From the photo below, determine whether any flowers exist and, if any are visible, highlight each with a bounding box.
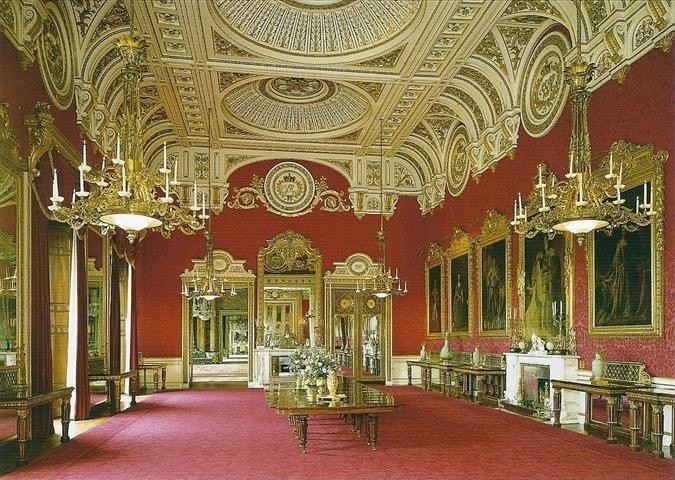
[285,334,344,380]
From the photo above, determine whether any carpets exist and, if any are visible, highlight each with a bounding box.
[1,388,675,480]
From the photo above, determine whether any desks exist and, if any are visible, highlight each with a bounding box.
[139,361,168,393]
[406,359,507,404]
[91,369,139,413]
[550,378,675,460]
[0,384,76,467]
[260,374,407,454]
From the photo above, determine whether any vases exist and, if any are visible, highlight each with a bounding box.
[440,339,455,360]
[591,351,606,380]
[419,345,427,359]
[325,373,339,397]
[472,347,481,367]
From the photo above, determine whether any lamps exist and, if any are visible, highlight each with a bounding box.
[353,116,410,300]
[47,0,209,244]
[178,106,240,302]
[507,0,659,240]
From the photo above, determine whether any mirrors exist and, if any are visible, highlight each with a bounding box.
[178,229,393,381]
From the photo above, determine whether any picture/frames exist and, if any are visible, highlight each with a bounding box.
[585,138,669,339]
[444,226,476,339]
[516,163,577,342]
[475,209,514,341]
[425,240,447,340]
